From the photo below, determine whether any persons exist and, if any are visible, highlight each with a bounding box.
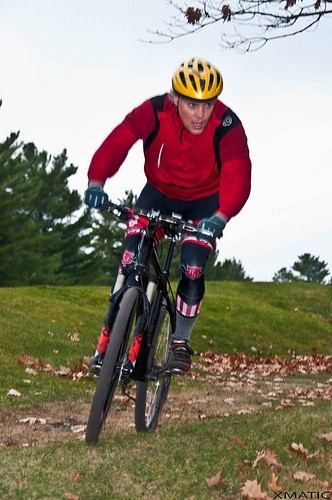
[85,57,251,376]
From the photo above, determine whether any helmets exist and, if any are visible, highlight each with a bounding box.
[172,57,222,103]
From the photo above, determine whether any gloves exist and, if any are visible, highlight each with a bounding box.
[196,215,226,240]
[84,185,108,208]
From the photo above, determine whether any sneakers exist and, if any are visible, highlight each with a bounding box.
[91,351,105,373]
[168,339,193,374]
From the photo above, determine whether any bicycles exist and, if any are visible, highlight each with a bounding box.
[81,197,218,448]
